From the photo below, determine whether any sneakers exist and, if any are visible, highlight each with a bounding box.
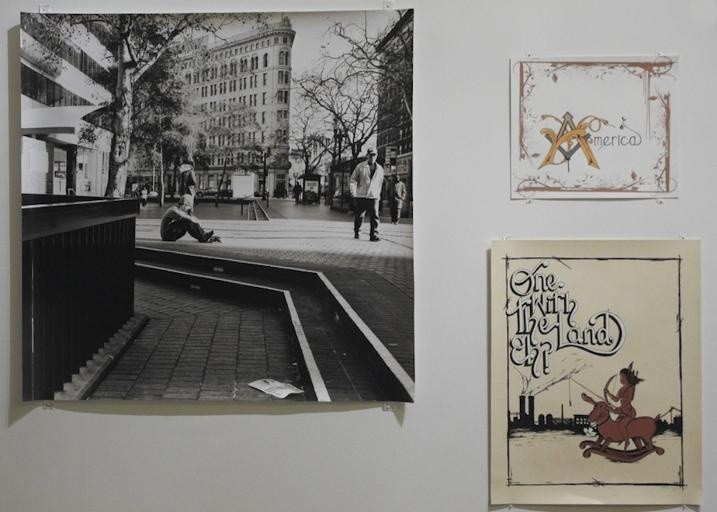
[370,238,380,241]
[354,234,359,238]
[199,230,213,243]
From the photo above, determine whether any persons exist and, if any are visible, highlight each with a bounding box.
[293,182,301,203]
[160,194,222,244]
[605,367,635,450]
[387,175,406,224]
[349,147,383,240]
[175,160,195,214]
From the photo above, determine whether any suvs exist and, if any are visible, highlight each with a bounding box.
[134,181,161,197]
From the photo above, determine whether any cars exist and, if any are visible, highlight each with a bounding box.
[198,186,232,198]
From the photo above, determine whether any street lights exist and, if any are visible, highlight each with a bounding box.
[153,114,167,206]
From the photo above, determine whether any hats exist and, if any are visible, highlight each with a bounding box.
[367,148,377,154]
